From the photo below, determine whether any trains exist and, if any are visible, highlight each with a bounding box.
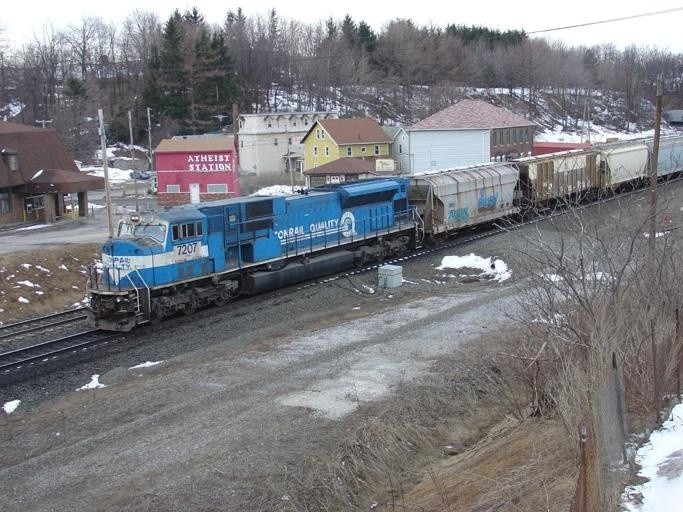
[81,130,683,332]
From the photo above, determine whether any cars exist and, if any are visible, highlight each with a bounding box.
[129,169,150,180]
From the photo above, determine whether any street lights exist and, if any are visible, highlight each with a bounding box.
[35,117,54,128]
